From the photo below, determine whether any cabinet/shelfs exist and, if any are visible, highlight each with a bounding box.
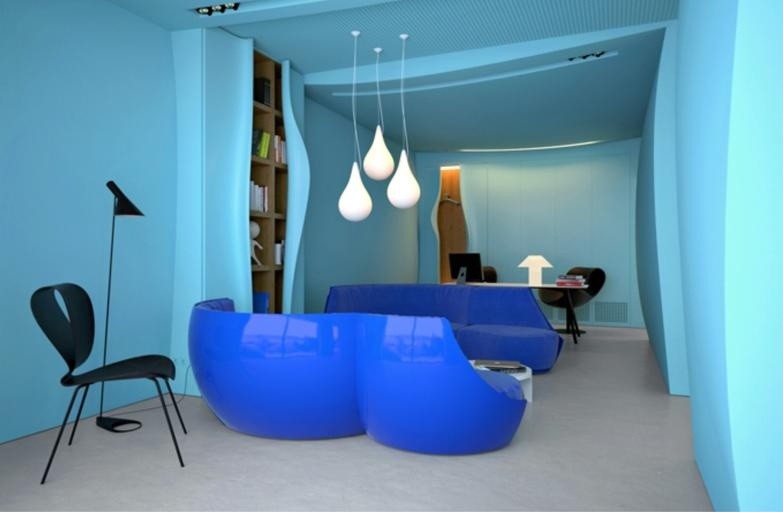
[249,50,288,313]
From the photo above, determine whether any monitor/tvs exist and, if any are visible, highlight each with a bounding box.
[448,252,482,285]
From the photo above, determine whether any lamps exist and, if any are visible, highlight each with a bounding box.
[96,180,145,435]
[336,28,422,225]
[517,254,553,285]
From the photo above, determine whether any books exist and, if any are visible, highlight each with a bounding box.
[249,179,268,213]
[252,128,286,164]
[555,274,587,288]
[471,358,526,375]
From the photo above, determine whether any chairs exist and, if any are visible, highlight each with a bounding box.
[30,283,188,489]
[538,268,606,334]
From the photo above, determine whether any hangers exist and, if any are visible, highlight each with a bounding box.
[435,191,461,207]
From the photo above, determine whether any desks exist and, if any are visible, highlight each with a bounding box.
[443,282,588,346]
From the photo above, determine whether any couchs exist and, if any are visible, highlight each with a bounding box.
[323,284,561,372]
[189,297,363,439]
[354,312,527,456]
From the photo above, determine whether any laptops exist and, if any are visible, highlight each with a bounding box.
[474,359,521,370]
